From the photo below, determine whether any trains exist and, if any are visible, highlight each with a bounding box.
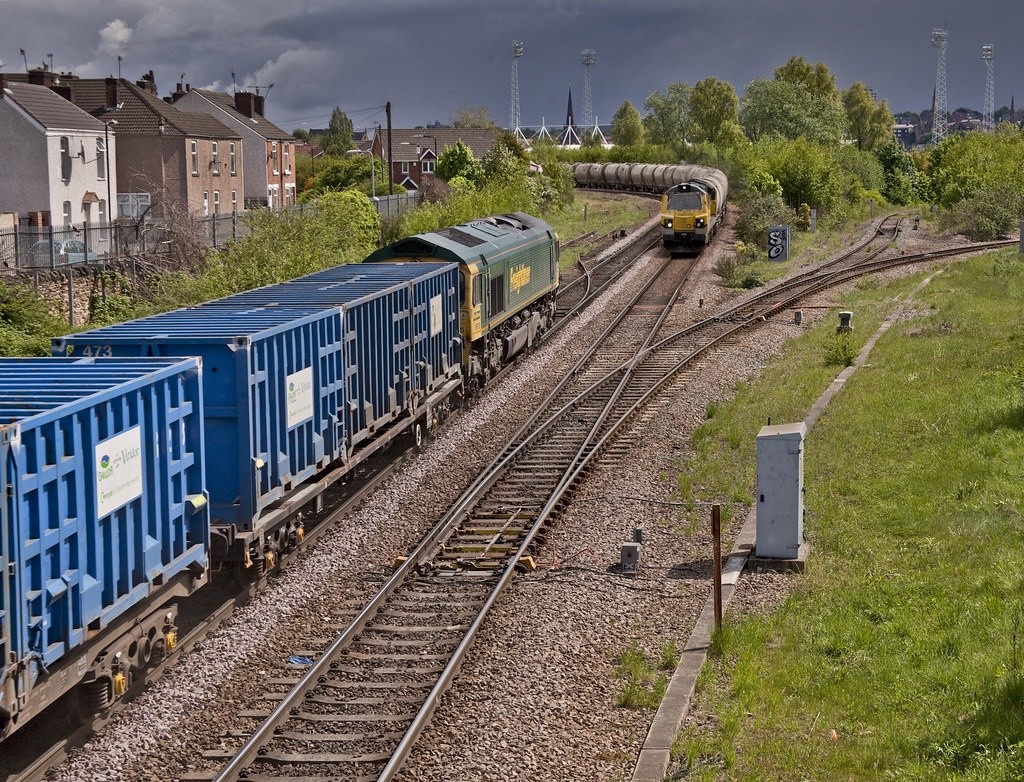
[0,210,562,744]
[569,161,729,256]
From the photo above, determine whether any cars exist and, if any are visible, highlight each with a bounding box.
[25,238,98,279]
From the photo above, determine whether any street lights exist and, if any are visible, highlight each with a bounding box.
[401,142,420,188]
[411,134,438,179]
[304,144,314,190]
[347,150,379,206]
[105,119,118,263]
